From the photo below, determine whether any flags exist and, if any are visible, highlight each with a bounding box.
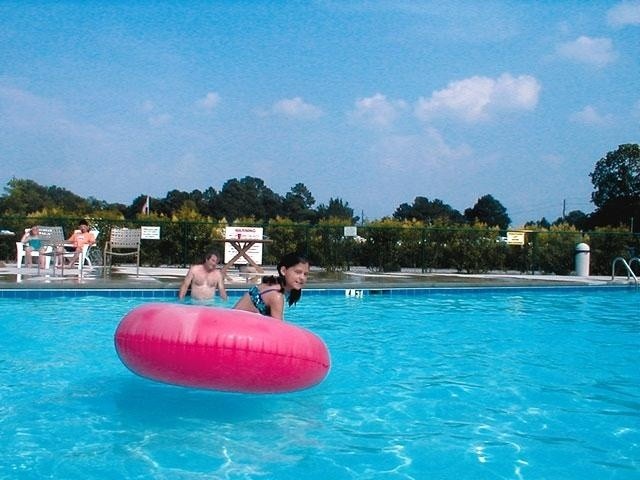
[142,195,149,214]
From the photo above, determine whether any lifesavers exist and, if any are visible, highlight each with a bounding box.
[115,303,330,392]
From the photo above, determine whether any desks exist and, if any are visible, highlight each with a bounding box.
[40,241,73,278]
[212,239,273,273]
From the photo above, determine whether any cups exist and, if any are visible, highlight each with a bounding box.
[237,233,241,239]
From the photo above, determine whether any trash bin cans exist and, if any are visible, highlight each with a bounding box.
[574,243,591,277]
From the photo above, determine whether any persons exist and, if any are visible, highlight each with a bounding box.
[231,251,312,322]
[55,220,96,269]
[20,224,48,269]
[179,251,227,301]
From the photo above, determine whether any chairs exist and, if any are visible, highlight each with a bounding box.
[58,230,100,269]
[15,229,53,269]
[104,227,142,278]
